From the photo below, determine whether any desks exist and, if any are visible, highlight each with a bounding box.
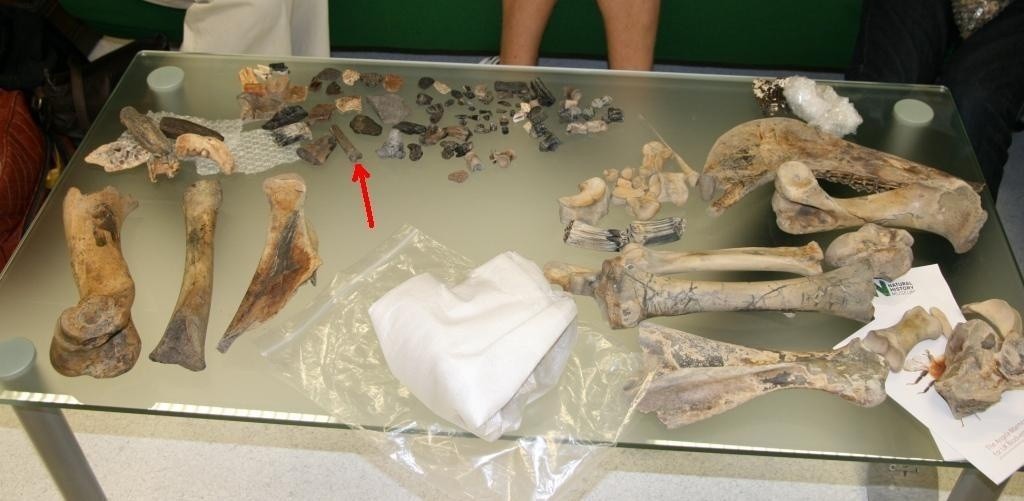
[0,50,1024,501]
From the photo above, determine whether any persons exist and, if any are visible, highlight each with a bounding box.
[142,1,331,57]
[477,0,661,72]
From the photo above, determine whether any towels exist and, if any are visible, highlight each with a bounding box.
[370,254,577,440]
